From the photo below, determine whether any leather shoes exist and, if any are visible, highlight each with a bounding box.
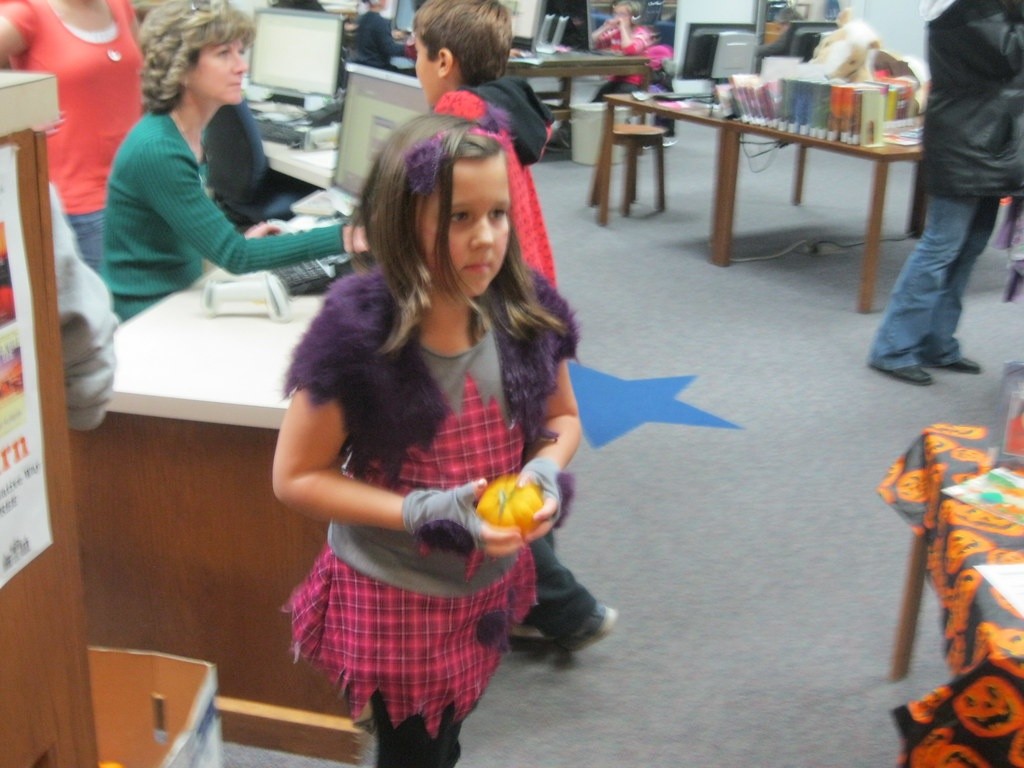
[870,364,933,386]
[938,358,980,374]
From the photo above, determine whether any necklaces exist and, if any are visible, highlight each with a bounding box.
[107,47,121,61]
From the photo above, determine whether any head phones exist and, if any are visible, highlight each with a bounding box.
[631,12,641,24]
[370,0,379,5]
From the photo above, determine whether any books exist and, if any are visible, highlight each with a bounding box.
[714,73,917,148]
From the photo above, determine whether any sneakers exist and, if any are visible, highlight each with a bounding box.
[557,603,619,651]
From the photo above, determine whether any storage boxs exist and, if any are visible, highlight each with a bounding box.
[85,643,219,768]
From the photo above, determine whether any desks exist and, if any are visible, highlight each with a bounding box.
[66,213,375,768]
[598,94,929,314]
[876,421,1024,768]
[339,22,653,156]
[234,74,343,192]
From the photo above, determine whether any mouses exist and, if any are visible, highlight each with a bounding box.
[631,90,650,100]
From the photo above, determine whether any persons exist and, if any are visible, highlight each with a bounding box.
[411,0,619,658]
[356,0,407,72]
[0,0,143,276]
[99,0,367,323]
[759,6,803,57]
[270,112,583,768]
[591,0,657,102]
[864,0,1024,385]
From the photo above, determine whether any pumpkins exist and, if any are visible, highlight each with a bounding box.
[477,472,545,537]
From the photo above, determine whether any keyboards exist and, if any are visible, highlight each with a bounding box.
[252,118,308,149]
[592,50,615,56]
[269,257,335,295]
[654,94,713,101]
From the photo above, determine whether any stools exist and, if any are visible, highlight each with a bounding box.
[588,123,668,219]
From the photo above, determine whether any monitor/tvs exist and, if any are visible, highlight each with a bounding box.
[331,63,430,208]
[537,0,592,54]
[785,20,839,61]
[677,22,756,89]
[249,7,344,108]
[393,0,418,35]
